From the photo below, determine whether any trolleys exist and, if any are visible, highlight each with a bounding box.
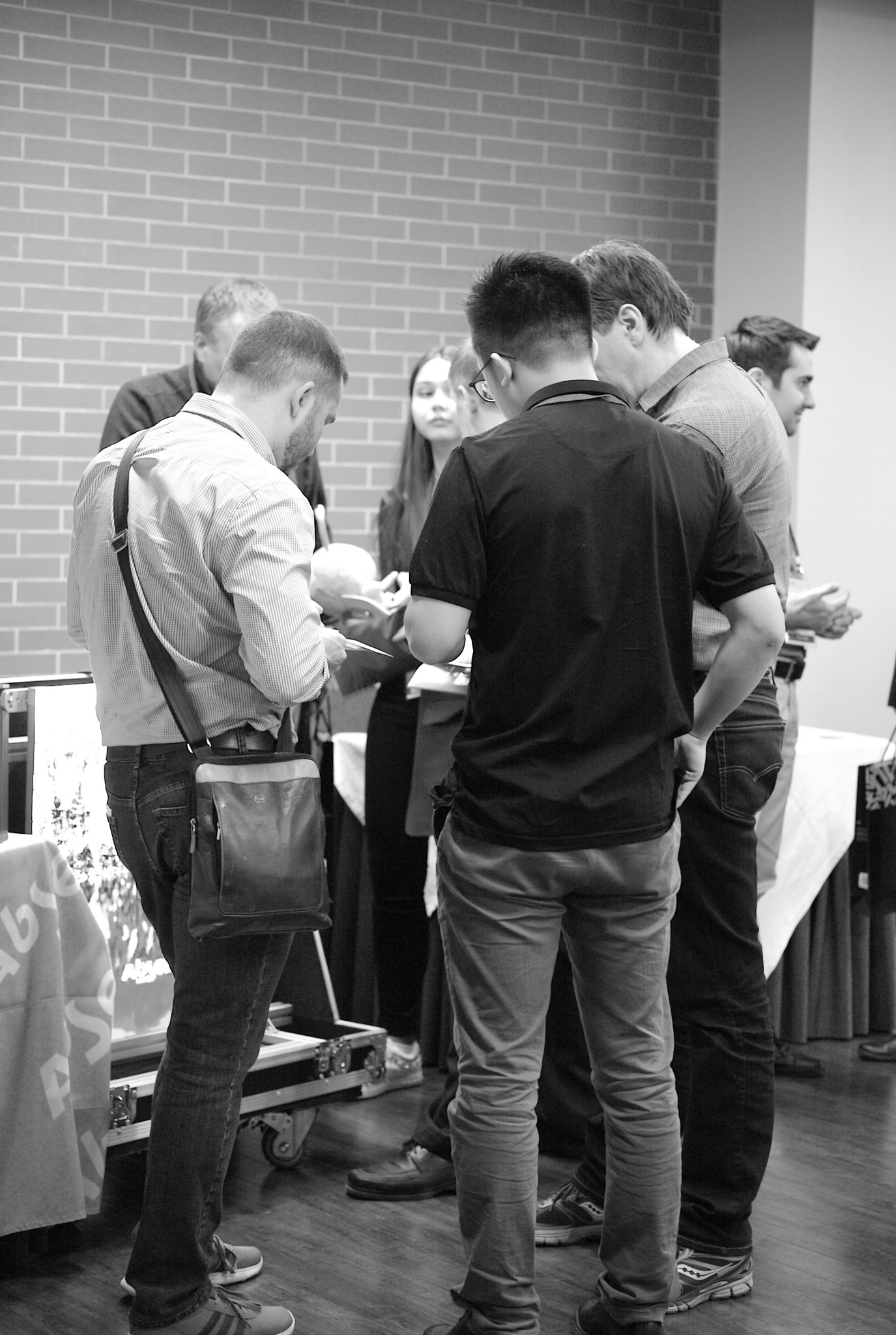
[0,672,387,1252]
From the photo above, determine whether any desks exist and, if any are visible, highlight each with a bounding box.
[329,727,896,1076]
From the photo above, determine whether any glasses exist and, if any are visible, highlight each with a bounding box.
[468,352,517,403]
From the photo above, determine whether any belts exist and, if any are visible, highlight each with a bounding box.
[774,660,805,680]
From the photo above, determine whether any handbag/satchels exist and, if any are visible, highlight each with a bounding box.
[849,729,896,920]
[183,747,332,938]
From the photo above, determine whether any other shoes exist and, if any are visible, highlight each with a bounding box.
[358,1039,423,1101]
[422,1317,470,1335]
[576,1295,667,1335]
[776,1053,825,1077]
[859,1032,896,1061]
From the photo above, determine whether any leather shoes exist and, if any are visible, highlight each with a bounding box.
[347,1140,458,1202]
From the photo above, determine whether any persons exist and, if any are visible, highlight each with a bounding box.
[534,241,790,1315]
[338,344,593,1204]
[711,311,871,1080]
[856,653,896,1061]
[96,267,362,1023]
[68,309,364,1335]
[396,250,790,1335]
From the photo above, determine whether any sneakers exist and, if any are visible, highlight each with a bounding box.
[535,1180,603,1244]
[121,1233,264,1296]
[130,1285,296,1334]
[666,1246,754,1316]
